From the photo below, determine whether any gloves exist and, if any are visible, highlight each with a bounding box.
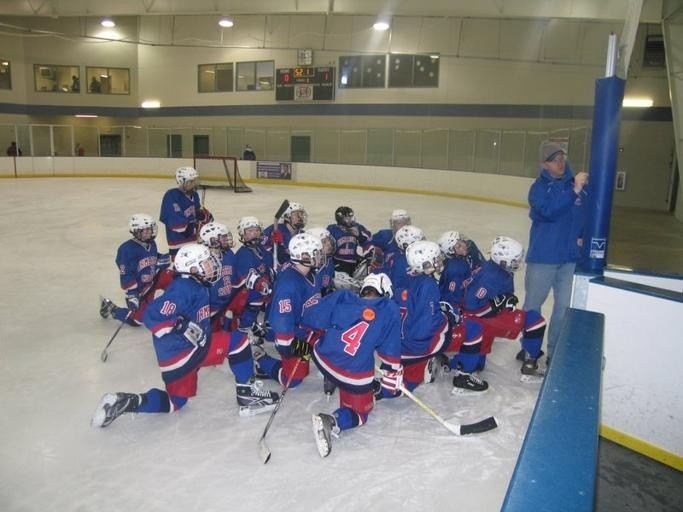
[488,292,520,313]
[186,222,203,234]
[294,338,314,362]
[441,308,465,328]
[377,363,405,394]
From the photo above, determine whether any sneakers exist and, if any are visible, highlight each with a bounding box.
[238,323,272,346]
[423,353,448,384]
[451,371,489,398]
[126,290,142,309]
[97,296,114,318]
[310,411,340,459]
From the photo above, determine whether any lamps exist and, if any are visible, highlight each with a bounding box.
[218,16,233,27]
[373,15,390,30]
[101,16,115,27]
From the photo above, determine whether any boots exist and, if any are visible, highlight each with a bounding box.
[232,380,281,417]
[322,375,337,402]
[89,388,142,429]
[250,344,267,378]
[372,378,382,400]
[520,351,545,384]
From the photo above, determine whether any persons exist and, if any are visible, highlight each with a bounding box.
[302,270,403,457]
[160,166,214,262]
[281,164,290,179]
[199,203,546,396]
[75,143,80,156]
[93,243,280,427]
[244,145,256,160]
[7,142,22,156]
[100,214,178,326]
[89,77,101,93]
[71,76,80,92]
[516,145,589,368]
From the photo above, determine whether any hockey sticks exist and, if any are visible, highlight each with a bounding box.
[257,332,313,464]
[100,286,150,360]
[272,199,290,270]
[401,383,498,437]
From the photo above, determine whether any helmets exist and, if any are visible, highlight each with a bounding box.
[403,240,440,275]
[306,228,337,258]
[174,167,198,192]
[127,212,159,243]
[394,225,427,254]
[437,232,462,258]
[198,222,234,250]
[488,235,525,273]
[358,274,395,299]
[281,202,307,229]
[287,232,327,273]
[334,206,355,228]
[173,242,222,287]
[236,216,262,239]
[389,209,411,228]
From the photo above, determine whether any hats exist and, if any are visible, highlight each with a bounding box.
[539,140,568,160]
